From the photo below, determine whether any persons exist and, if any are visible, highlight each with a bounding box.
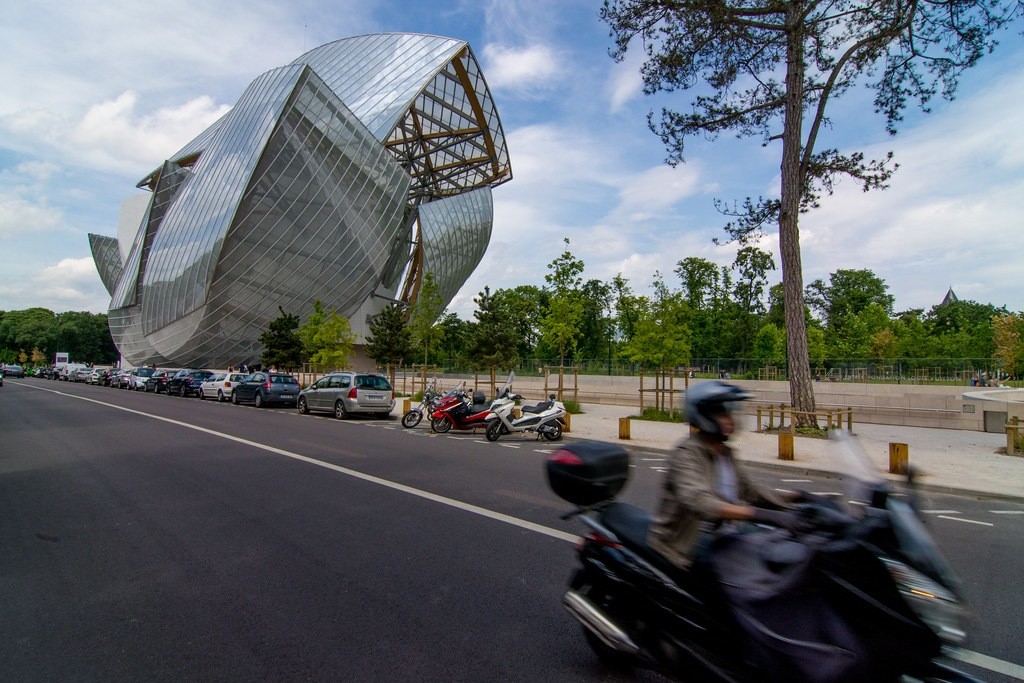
[227,363,278,374]
[71,360,94,368]
[111,360,117,368]
[143,362,149,368]
[815,370,821,382]
[643,378,817,575]
[718,370,731,380]
[970,373,1011,388]
[689,369,695,379]
[674,366,679,378]
[701,363,708,373]
[152,363,156,371]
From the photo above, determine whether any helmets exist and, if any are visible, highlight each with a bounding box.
[684,381,757,441]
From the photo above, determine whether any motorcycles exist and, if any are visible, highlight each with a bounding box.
[401,377,566,441]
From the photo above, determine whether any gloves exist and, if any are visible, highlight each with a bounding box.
[796,489,839,510]
[755,509,807,541]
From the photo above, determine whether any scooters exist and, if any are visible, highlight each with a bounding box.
[549,443,984,682]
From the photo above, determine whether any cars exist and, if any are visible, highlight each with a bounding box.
[0,362,395,419]
